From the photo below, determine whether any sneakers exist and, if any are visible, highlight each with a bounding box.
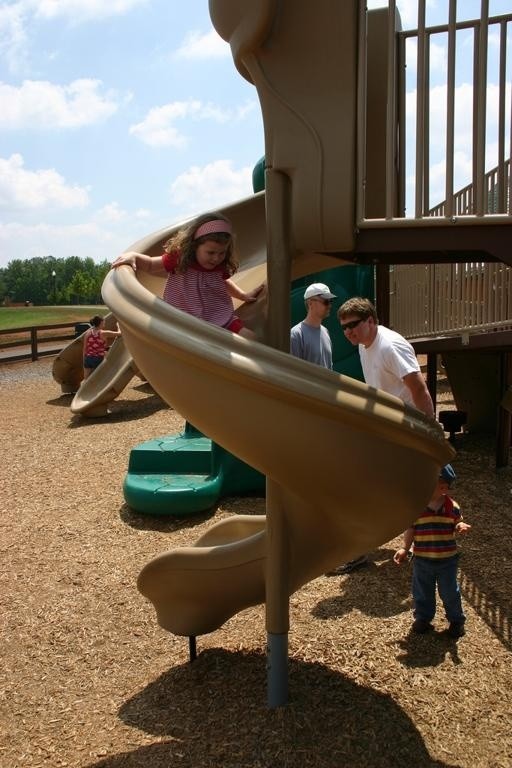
[412,620,430,633]
[331,554,368,574]
[449,622,464,637]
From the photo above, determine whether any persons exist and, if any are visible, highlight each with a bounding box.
[290,283,337,371]
[325,297,434,578]
[111,212,264,342]
[394,466,473,636]
[82,314,121,380]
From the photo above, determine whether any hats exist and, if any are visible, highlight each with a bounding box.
[304,283,337,299]
[440,463,455,484]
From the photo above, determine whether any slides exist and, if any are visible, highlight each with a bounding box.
[52,312,119,395]
[69,332,147,420]
[99,194,445,640]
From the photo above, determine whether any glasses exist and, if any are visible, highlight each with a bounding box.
[340,319,362,330]
[311,298,333,306]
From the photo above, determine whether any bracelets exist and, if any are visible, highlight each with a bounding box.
[401,546,408,552]
